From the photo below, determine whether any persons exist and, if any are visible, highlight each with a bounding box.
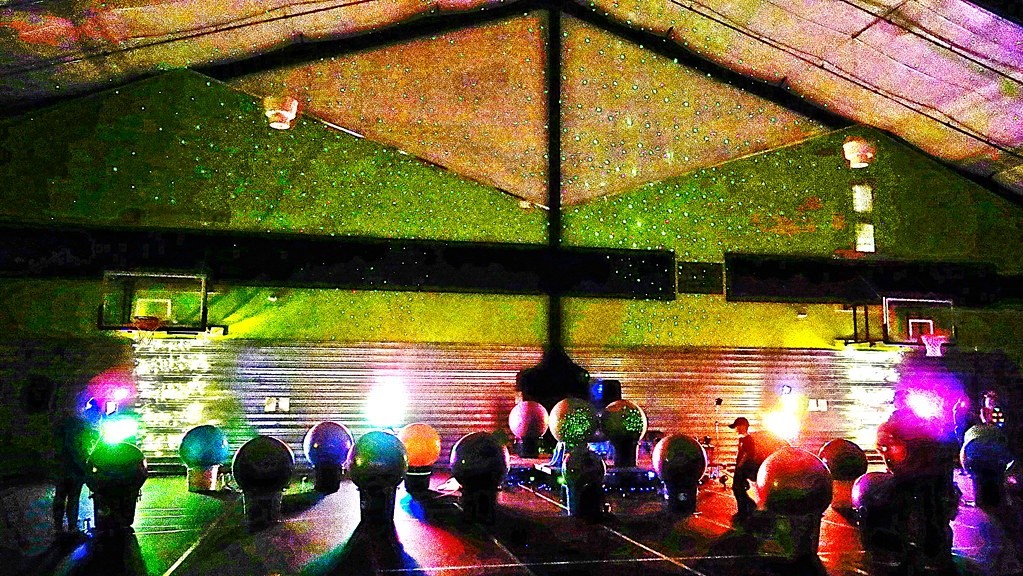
[888,389,921,430]
[47,374,102,537]
[728,417,757,520]
[952,391,1008,475]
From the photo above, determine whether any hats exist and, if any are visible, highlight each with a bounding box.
[728,417,750,429]
